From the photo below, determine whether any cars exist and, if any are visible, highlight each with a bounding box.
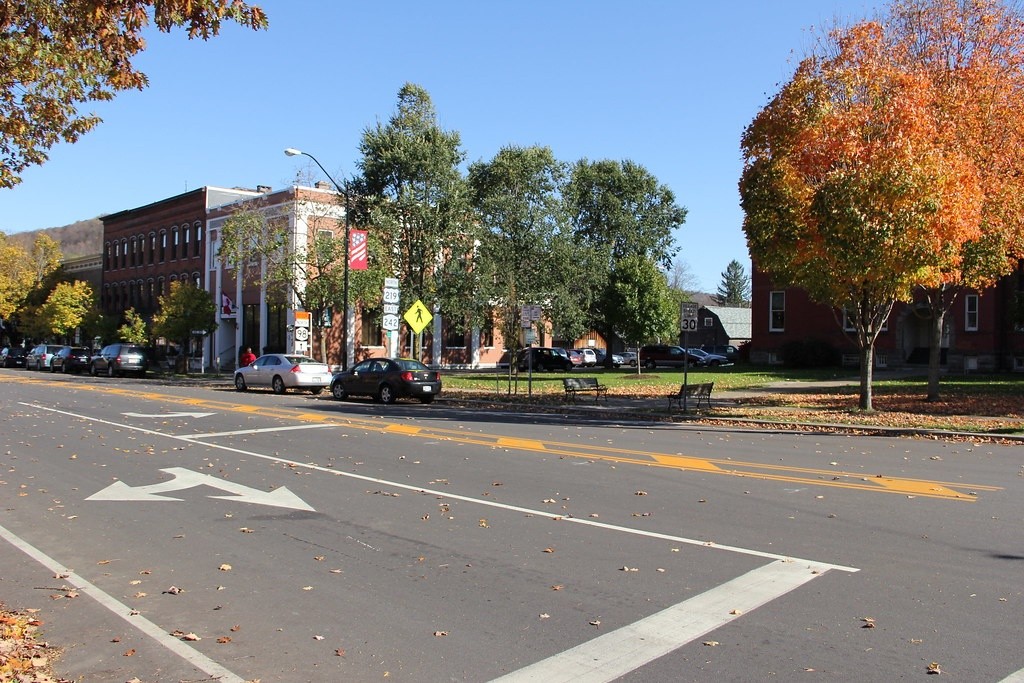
[538,348,638,368]
[518,347,574,373]
[233,354,333,395]
[26,344,67,371]
[330,358,442,405]
[677,349,729,366]
[0,347,28,368]
[89,344,149,378]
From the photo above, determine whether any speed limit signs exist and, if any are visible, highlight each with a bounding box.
[681,302,698,332]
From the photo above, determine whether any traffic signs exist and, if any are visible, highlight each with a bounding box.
[191,329,208,336]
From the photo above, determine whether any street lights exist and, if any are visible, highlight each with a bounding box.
[285,148,349,372]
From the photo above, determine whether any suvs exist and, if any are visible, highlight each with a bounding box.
[50,346,92,374]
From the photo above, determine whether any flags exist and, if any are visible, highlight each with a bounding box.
[222,292,232,315]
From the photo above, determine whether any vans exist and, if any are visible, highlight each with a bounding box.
[638,345,707,369]
[701,345,738,363]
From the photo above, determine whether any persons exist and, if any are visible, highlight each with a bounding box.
[241,348,256,367]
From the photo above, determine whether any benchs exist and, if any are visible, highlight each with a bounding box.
[666,382,715,409]
[561,377,610,403]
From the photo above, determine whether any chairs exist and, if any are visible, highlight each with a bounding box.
[376,363,383,372]
